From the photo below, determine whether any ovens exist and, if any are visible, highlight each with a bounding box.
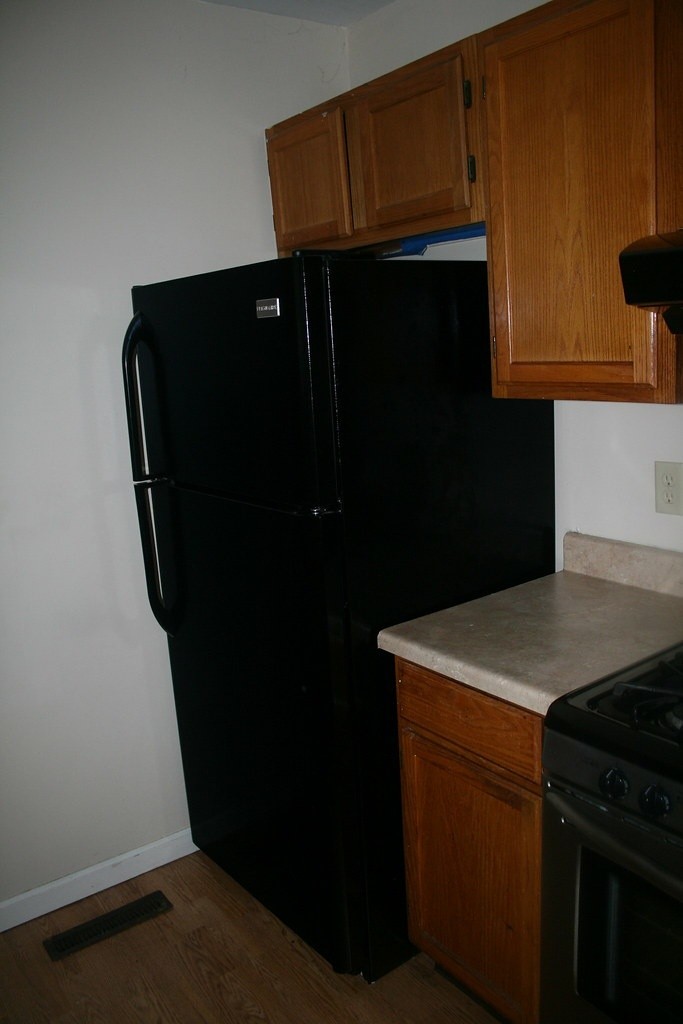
[538,785,683,1024]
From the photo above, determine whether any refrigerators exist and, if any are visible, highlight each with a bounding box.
[123,254,553,988]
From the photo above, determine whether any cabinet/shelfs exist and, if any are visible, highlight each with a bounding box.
[396,656,544,1024]
[268,0,683,405]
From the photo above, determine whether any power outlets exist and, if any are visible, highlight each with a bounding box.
[655,460,683,516]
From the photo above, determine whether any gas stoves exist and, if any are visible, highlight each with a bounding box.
[542,640,683,837]
